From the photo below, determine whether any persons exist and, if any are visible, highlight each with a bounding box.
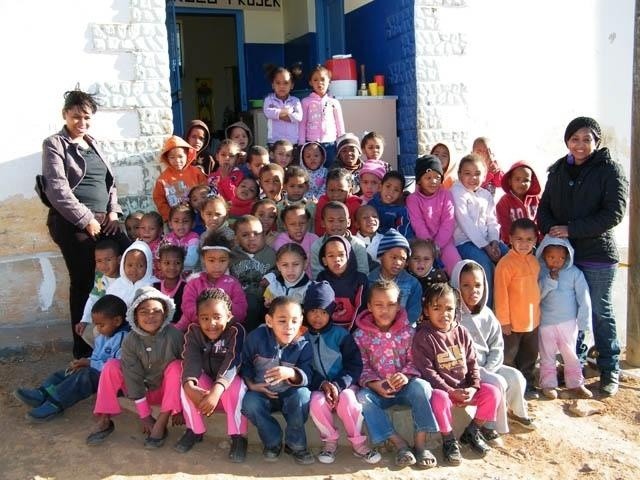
[35,90,133,359]
[537,117,628,395]
[12,294,131,422]
[291,65,310,90]
[535,233,593,398]
[75,119,542,467]
[263,68,303,166]
[297,66,346,169]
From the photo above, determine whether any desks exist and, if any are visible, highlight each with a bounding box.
[248,94,400,170]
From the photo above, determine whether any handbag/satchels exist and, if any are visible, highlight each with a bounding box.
[35,175,52,208]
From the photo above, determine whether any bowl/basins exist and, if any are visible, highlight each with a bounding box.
[249,99,264,108]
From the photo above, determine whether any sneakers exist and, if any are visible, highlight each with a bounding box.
[526,368,618,399]
[229,437,247,463]
[144,427,168,448]
[285,444,315,464]
[263,441,282,462]
[88,420,114,445]
[174,429,203,452]
[318,441,338,464]
[26,400,60,423]
[352,444,382,464]
[13,387,45,408]
[395,411,538,468]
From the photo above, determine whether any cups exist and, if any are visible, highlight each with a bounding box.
[374,75,384,96]
[369,82,378,95]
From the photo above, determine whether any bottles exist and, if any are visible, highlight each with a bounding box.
[359,64,368,96]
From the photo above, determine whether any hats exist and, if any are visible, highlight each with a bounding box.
[359,159,387,179]
[336,133,362,158]
[304,280,336,317]
[415,155,444,184]
[565,117,602,149]
[377,228,412,258]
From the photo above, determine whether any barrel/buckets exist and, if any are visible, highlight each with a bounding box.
[325,58,358,98]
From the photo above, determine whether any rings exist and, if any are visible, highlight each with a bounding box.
[557,230,561,235]
